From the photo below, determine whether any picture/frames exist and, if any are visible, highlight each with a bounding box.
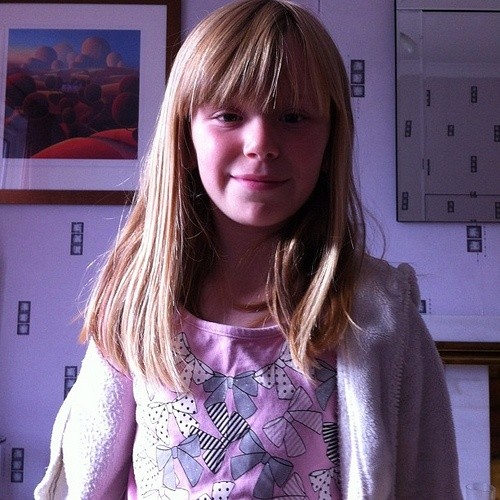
[0,0,184,208]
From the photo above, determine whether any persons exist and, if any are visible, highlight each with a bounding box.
[32,1,462,500]
